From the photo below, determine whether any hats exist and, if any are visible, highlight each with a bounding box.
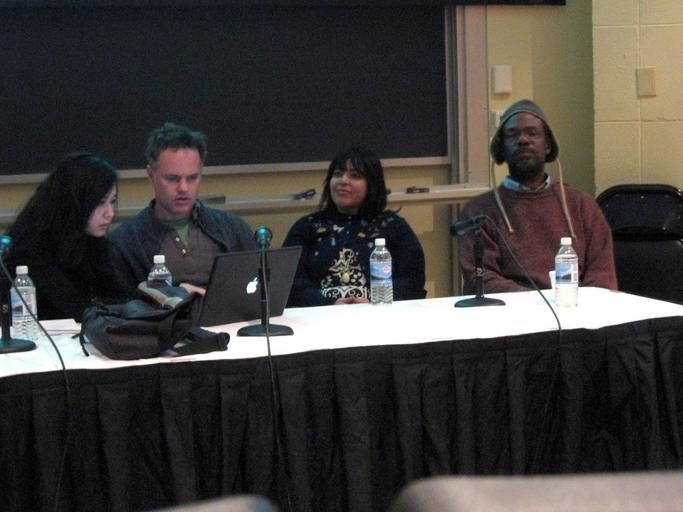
[489,100,561,165]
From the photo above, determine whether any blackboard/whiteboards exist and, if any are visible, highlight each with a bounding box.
[0,1,454,184]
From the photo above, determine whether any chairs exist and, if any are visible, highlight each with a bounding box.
[596,183,682,305]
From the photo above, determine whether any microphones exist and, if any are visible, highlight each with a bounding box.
[451,215,489,235]
[1,233,14,261]
[254,225,274,250]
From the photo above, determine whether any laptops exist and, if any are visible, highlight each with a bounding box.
[195,246,303,326]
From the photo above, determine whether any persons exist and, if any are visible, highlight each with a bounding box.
[0,153,154,316]
[457,100,618,294]
[104,123,270,305]
[281,145,424,308]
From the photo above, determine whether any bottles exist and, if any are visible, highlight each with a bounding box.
[553,236,578,308]
[147,255,172,289]
[370,238,394,304]
[10,265,39,344]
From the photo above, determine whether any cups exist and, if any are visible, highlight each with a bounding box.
[549,270,557,296]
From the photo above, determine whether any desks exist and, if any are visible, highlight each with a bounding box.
[0,285,681,512]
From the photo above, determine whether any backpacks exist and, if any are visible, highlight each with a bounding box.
[79,278,229,361]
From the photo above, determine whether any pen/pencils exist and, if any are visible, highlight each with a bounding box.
[408,188,430,192]
